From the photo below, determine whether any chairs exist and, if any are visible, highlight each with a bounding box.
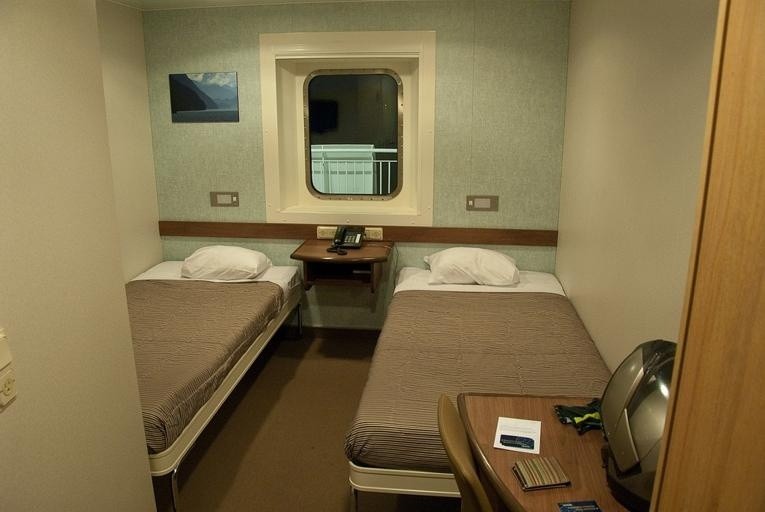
[437,392,502,512]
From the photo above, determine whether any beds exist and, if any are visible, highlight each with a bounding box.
[346,267,614,512]
[125,261,302,512]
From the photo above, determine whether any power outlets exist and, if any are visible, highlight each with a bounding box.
[316,226,337,239]
[0,370,17,408]
[364,227,383,240]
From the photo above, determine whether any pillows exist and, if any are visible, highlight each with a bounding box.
[180,245,270,283]
[425,248,518,287]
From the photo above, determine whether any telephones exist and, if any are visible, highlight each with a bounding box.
[333,225,364,249]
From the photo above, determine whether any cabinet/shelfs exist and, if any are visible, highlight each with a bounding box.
[649,0,765,511]
[289,239,395,294]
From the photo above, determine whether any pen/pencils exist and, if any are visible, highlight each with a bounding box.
[558,500,602,512]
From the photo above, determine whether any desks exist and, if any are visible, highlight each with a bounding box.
[457,390,629,512]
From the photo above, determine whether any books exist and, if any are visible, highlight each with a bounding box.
[510,455,571,492]
